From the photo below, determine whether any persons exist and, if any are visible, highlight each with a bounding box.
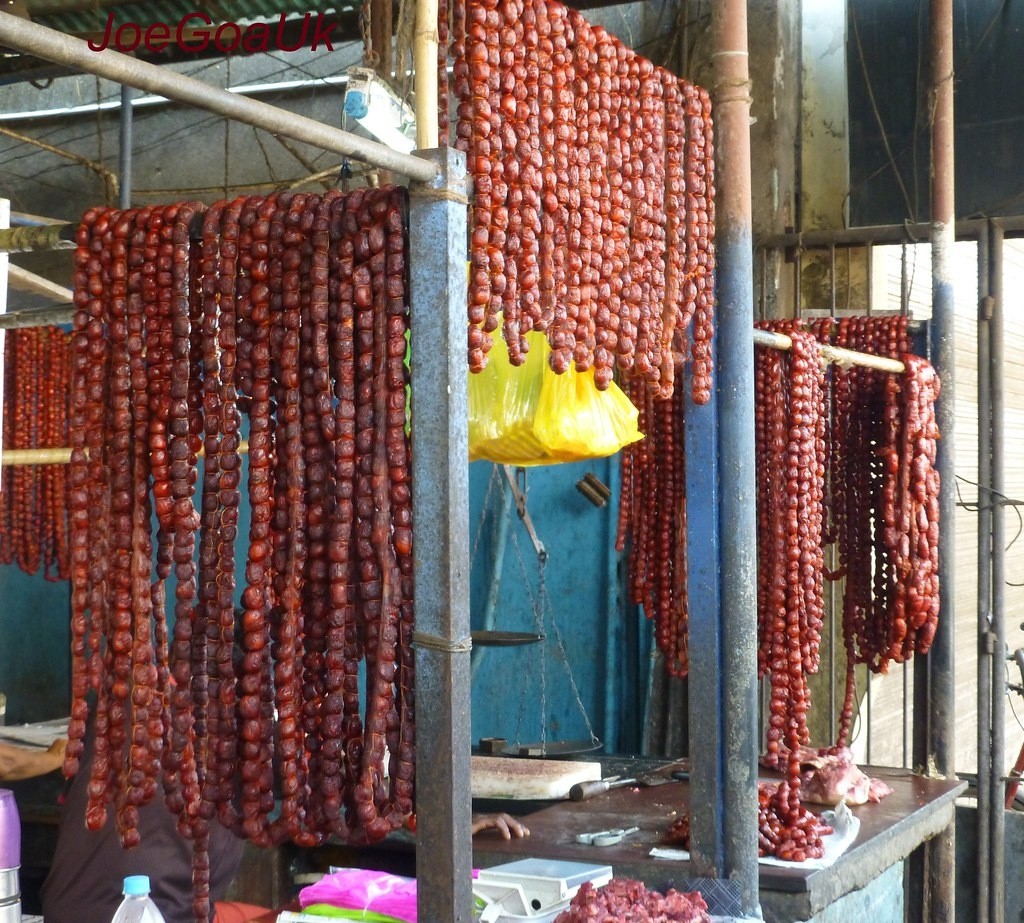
[42,655,532,923]
[0,722,73,783]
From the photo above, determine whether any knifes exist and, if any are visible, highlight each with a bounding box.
[569,772,680,801]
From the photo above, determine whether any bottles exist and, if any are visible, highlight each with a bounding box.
[111,875,166,923]
